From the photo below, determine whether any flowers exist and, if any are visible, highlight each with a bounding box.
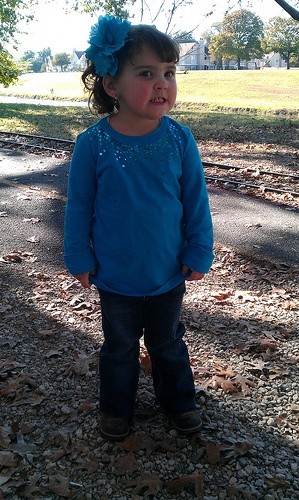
[85,14,132,77]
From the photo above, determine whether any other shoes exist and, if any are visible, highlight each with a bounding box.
[170,410,203,433]
[100,413,129,437]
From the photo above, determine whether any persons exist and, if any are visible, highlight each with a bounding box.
[62,23,214,441]
